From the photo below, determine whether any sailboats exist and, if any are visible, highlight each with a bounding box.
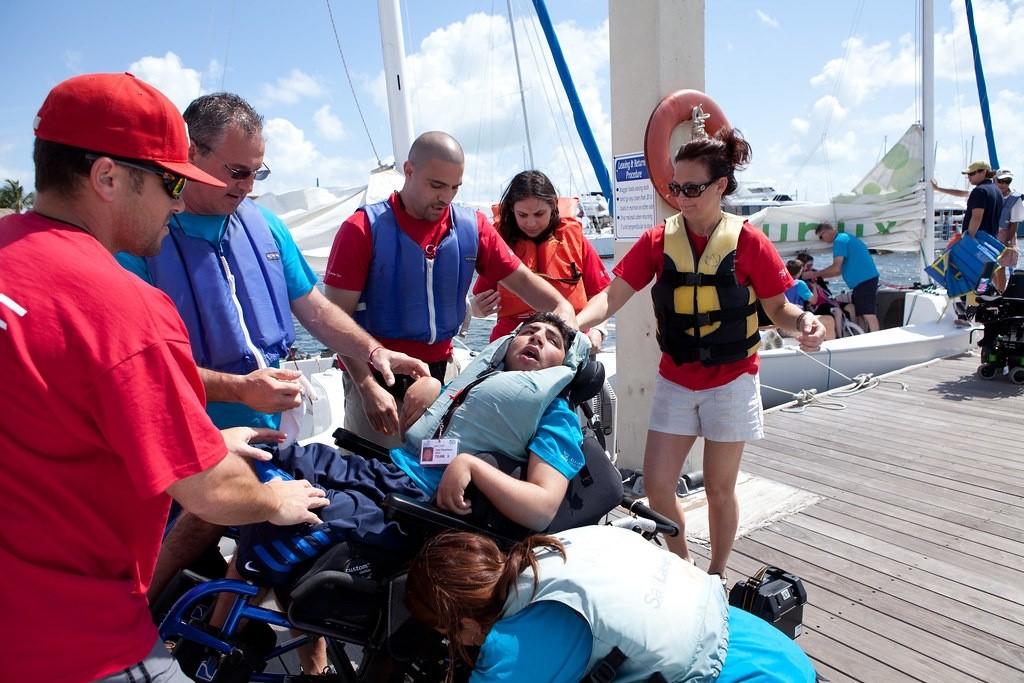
[277,0,986,410]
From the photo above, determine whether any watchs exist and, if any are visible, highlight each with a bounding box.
[590,326,608,343]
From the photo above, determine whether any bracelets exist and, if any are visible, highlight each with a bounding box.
[369,347,384,366]
[795,313,806,331]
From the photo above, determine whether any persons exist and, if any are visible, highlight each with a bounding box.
[0,72,329,683]
[575,125,827,586]
[954,161,1004,326]
[468,169,612,362]
[144,310,588,637]
[323,131,579,450]
[783,259,818,310]
[405,526,822,683]
[115,93,432,675]
[801,223,881,333]
[930,168,1024,295]
[794,252,843,339]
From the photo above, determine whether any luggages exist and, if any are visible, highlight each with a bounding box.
[729,567,807,640]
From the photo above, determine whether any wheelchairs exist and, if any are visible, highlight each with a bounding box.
[155,351,678,683]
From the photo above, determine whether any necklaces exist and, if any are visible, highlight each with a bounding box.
[31,210,90,235]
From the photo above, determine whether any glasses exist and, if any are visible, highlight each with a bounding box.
[997,179,1012,184]
[85,155,187,199]
[819,231,825,239]
[668,178,718,198]
[968,172,976,176]
[192,138,271,180]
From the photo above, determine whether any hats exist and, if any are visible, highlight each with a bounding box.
[33,71,228,188]
[962,161,989,174]
[993,166,1014,179]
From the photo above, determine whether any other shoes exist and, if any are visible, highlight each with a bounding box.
[690,558,696,567]
[706,570,728,598]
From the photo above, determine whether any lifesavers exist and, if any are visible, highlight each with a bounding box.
[643,88,738,213]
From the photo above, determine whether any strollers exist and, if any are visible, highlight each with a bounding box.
[975,271,1024,385]
